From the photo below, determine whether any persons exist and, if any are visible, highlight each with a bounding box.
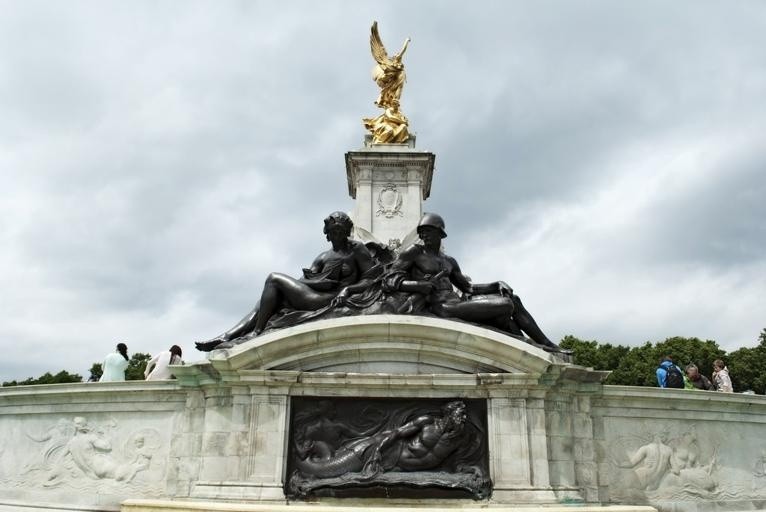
[383,211,576,356]
[656,355,684,388]
[375,54,410,143]
[301,397,469,478]
[195,211,376,351]
[711,360,733,393]
[602,423,766,501]
[712,365,729,391]
[99,343,129,382]
[142,345,183,379]
[743,386,755,394]
[22,416,151,486]
[681,363,715,390]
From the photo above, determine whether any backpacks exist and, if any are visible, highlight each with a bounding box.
[659,363,684,388]
